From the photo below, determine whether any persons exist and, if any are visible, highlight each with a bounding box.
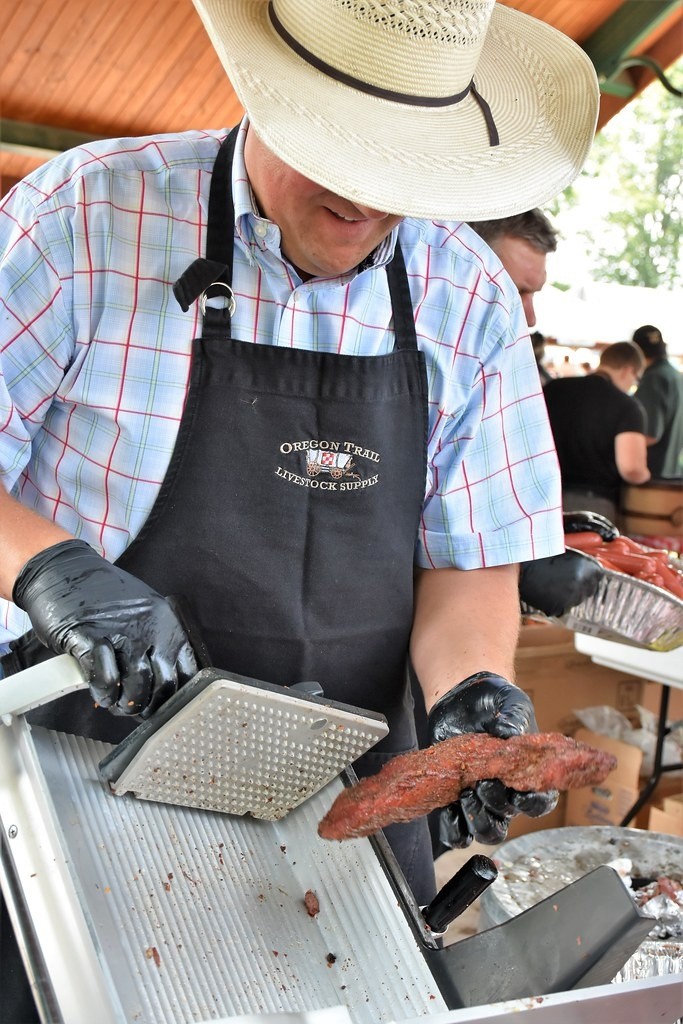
[464,207,683,529]
[0,0,583,1024]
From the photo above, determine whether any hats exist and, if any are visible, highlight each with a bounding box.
[634,325,664,359]
[193,0,603,222]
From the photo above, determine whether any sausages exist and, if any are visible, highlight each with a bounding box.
[563,509,683,607]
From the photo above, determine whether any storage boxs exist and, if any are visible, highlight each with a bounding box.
[567,727,683,835]
[508,625,683,838]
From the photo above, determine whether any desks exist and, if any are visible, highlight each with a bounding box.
[574,631,683,827]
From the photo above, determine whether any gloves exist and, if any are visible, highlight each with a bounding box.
[429,671,561,849]
[563,510,620,543]
[517,545,604,618]
[12,539,200,723]
